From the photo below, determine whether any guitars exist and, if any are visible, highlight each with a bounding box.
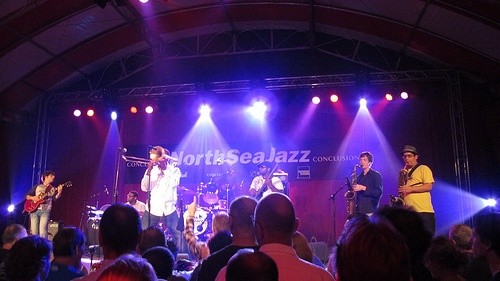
[24,180,73,212]
[253,163,278,199]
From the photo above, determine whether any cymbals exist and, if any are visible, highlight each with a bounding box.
[174,186,192,194]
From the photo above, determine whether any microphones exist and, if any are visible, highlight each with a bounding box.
[355,164,361,167]
[149,146,157,150]
[346,177,350,187]
[387,194,398,202]
[118,147,127,153]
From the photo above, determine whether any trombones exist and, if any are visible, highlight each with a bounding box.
[121,145,179,167]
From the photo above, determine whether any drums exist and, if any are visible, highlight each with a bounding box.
[218,198,229,210]
[88,210,105,221]
[201,184,221,205]
[183,208,212,235]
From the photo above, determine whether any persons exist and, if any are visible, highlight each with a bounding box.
[249,161,284,201]
[216,194,335,281]
[199,172,230,207]
[330,206,500,281]
[225,249,278,281]
[46,227,88,281]
[5,235,52,281]
[0,224,28,281]
[141,227,195,281]
[139,149,181,257]
[293,230,324,268]
[26,170,62,238]
[351,151,382,218]
[183,196,258,281]
[398,146,436,235]
[71,203,157,281]
[126,191,145,218]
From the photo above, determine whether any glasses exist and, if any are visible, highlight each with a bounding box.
[402,154,415,158]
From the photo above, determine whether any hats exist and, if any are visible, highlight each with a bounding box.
[401,144,417,155]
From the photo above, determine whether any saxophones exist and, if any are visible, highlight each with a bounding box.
[390,162,408,208]
[344,163,361,217]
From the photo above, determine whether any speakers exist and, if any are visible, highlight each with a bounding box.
[310,242,329,261]
[37,221,64,241]
